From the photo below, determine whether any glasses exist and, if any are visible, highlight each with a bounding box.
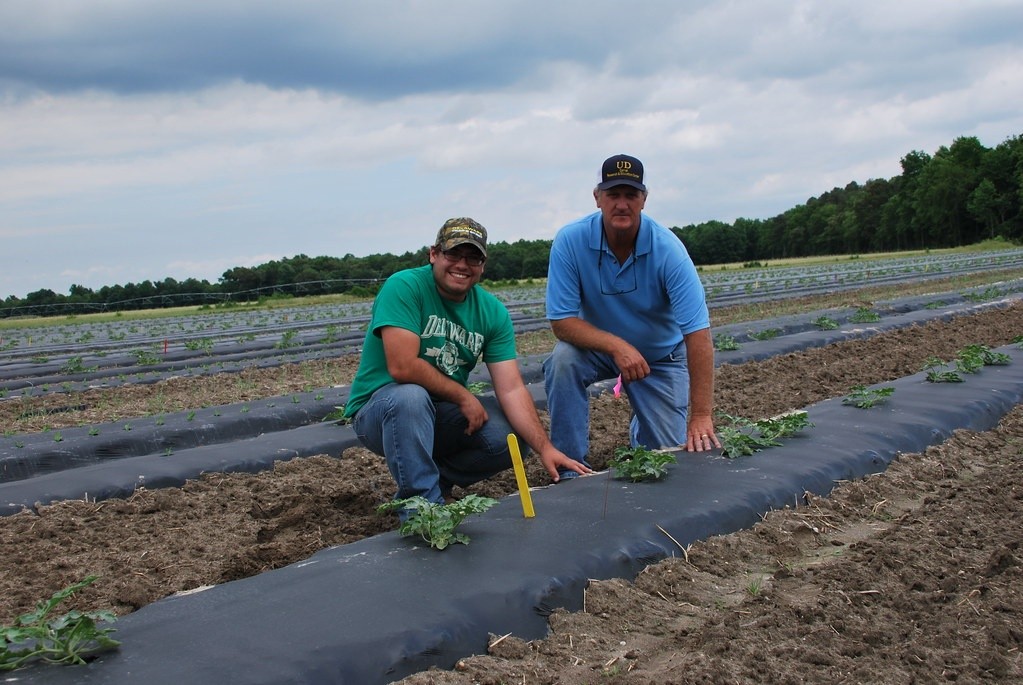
[598,262,637,295]
[443,249,484,268]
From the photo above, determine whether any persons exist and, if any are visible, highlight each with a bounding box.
[348,217,595,538]
[543,154,722,482]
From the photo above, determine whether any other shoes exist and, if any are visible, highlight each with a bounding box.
[440,480,454,500]
[550,478,574,485]
[629,410,635,422]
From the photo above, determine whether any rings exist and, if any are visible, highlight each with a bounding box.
[702,435,707,438]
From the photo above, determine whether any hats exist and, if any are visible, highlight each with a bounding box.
[434,217,487,256]
[597,155,646,192]
